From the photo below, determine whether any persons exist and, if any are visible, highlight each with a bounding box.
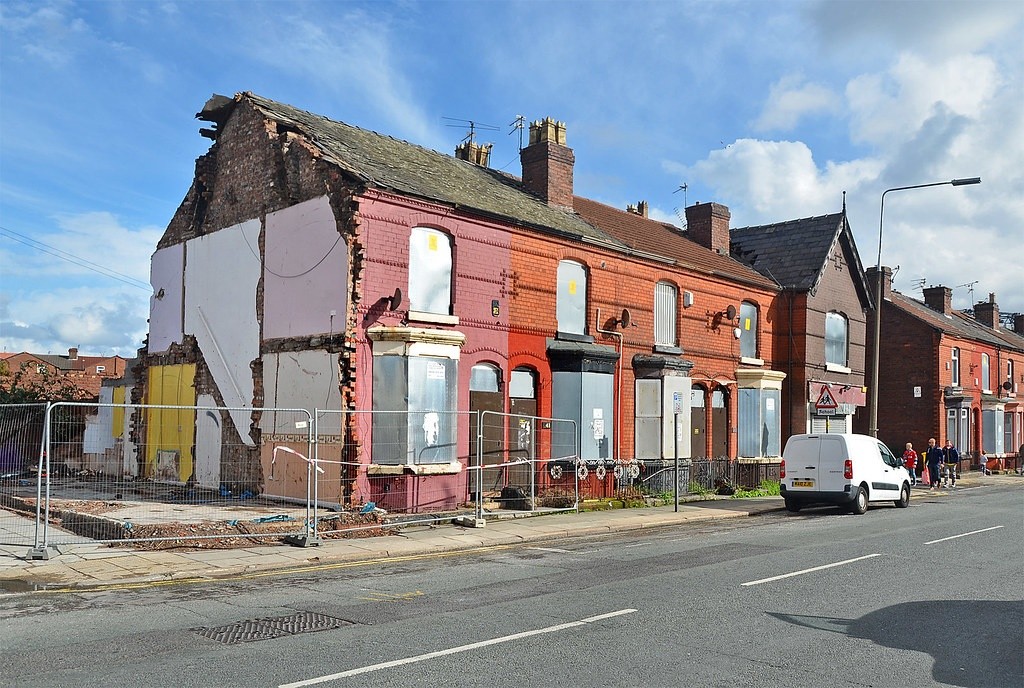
[942,440,960,488]
[902,443,918,486]
[925,438,944,491]
[980,450,988,476]
[1019,444,1024,475]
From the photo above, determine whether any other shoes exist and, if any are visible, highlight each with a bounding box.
[944,484,949,488]
[938,484,942,489]
[951,484,956,488]
[983,474,987,476]
[930,487,935,490]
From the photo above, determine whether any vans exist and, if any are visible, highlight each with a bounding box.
[778,432,912,515]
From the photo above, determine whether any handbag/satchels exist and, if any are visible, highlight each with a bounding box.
[922,468,930,484]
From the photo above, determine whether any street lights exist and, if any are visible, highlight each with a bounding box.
[870,177,982,437]
